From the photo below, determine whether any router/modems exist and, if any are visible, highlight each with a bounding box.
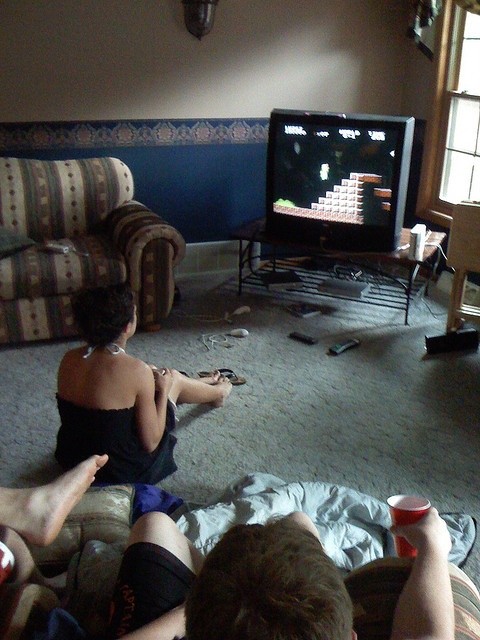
[261,268,304,292]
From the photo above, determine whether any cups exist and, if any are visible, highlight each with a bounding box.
[387,493,431,558]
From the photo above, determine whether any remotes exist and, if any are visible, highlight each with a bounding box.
[289,330,316,346]
[328,337,361,356]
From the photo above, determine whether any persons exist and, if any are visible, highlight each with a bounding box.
[0,455,207,640]
[55,285,234,485]
[171,505,480,640]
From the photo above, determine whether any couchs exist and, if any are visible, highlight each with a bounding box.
[0,155,188,347]
[0,488,479,640]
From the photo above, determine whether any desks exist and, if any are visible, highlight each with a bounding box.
[228,216,447,325]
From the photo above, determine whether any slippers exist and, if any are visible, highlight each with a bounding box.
[197,368,248,386]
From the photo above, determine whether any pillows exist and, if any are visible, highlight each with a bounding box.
[0,224,36,258]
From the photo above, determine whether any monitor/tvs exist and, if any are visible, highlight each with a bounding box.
[264,109,428,253]
